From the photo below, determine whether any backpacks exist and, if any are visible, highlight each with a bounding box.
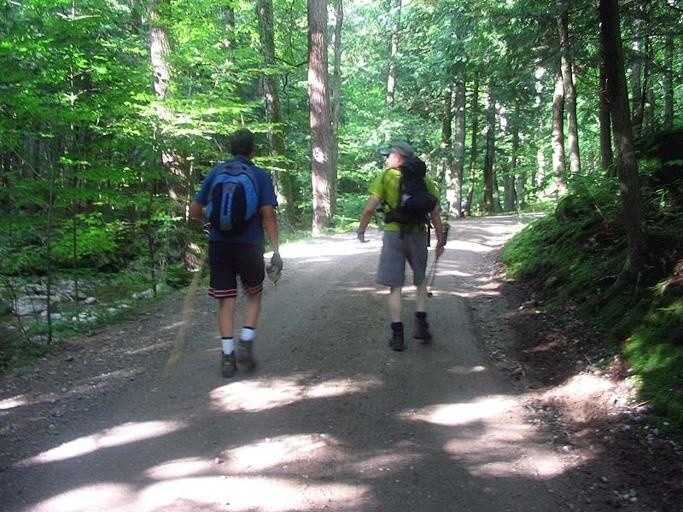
[385,156,438,223]
[204,159,262,237]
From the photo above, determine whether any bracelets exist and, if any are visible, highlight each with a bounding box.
[357,231,366,235]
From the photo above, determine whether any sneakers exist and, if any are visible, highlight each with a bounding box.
[235,338,258,371]
[413,318,432,342]
[220,348,236,379]
[386,332,404,351]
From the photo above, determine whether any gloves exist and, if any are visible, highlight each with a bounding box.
[265,250,284,276]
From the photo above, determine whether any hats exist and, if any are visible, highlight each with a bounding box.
[377,142,415,158]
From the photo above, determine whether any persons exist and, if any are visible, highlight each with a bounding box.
[358,143,444,351]
[191,128,282,377]
